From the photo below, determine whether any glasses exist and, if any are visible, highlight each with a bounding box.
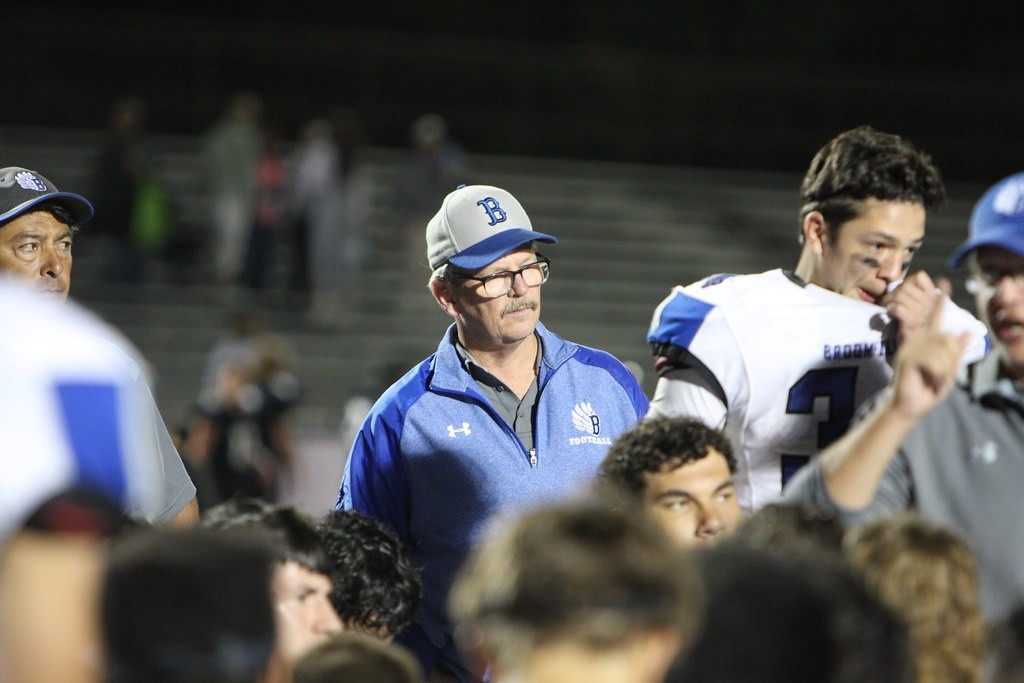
[452,252,551,298]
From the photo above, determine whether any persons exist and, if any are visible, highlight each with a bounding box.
[0,125,1024,683]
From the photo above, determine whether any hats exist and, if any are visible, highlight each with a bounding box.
[0,167,94,226]
[425,184,558,272]
[948,172,1023,269]
